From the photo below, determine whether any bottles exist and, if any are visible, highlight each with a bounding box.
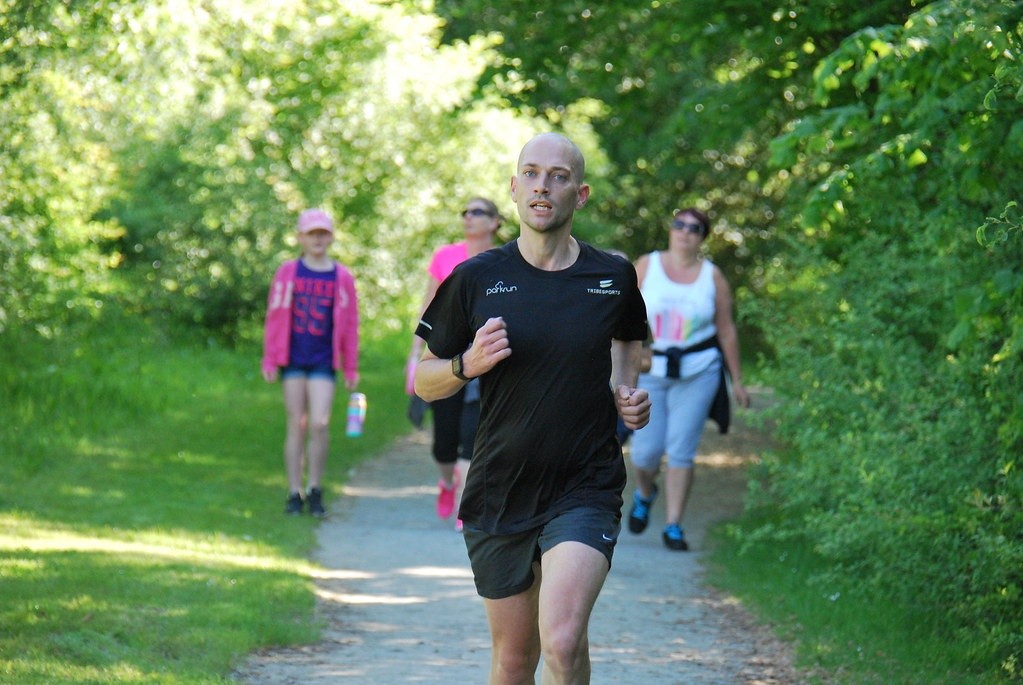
[346,392,368,438]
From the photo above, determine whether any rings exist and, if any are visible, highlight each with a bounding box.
[627,397,630,406]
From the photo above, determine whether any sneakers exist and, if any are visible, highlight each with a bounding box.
[284,492,302,519]
[454,517,465,533]
[627,481,660,535]
[304,489,327,518]
[437,469,462,518]
[659,523,688,552]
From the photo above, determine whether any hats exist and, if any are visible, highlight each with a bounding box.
[298,208,333,234]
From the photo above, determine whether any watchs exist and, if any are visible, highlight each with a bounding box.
[452,351,478,381]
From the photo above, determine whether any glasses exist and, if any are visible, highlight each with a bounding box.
[462,208,494,217]
[671,220,702,233]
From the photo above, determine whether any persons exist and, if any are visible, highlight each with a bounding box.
[606,209,748,549]
[262,209,359,518]
[413,132,652,685]
[411,198,501,532]
